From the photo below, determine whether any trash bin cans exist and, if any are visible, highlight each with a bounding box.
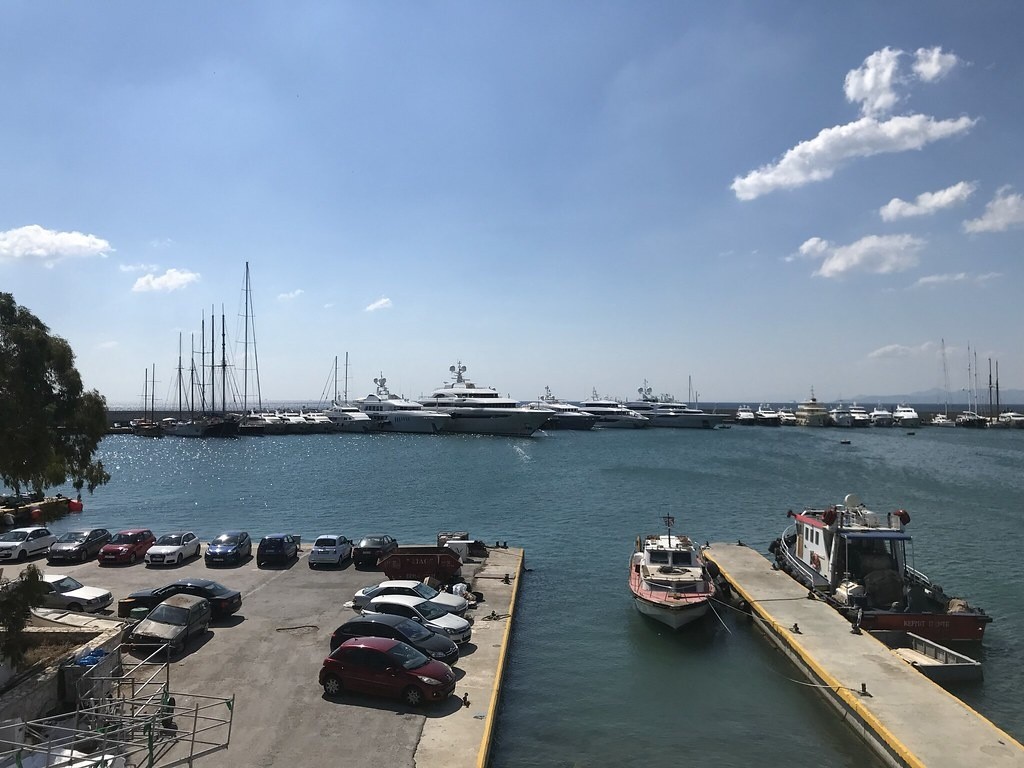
[60,650,115,707]
[376,547,462,584]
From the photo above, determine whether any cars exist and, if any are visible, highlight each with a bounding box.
[361,595,471,645]
[0,573,114,612]
[330,614,459,667]
[205,531,252,566]
[352,580,468,618]
[319,637,456,708]
[97,529,156,565]
[128,579,242,621]
[257,533,297,567]
[0,526,58,564]
[128,594,212,654]
[144,532,200,566]
[308,535,353,568]
[353,534,398,570]
[46,528,112,564]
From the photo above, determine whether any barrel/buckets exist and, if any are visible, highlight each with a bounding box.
[118,599,136,618]
[129,607,149,621]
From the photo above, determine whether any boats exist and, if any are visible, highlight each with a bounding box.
[27,605,140,643]
[628,512,716,630]
[769,494,993,677]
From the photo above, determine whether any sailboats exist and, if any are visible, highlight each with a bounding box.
[127,261,1024,440]
